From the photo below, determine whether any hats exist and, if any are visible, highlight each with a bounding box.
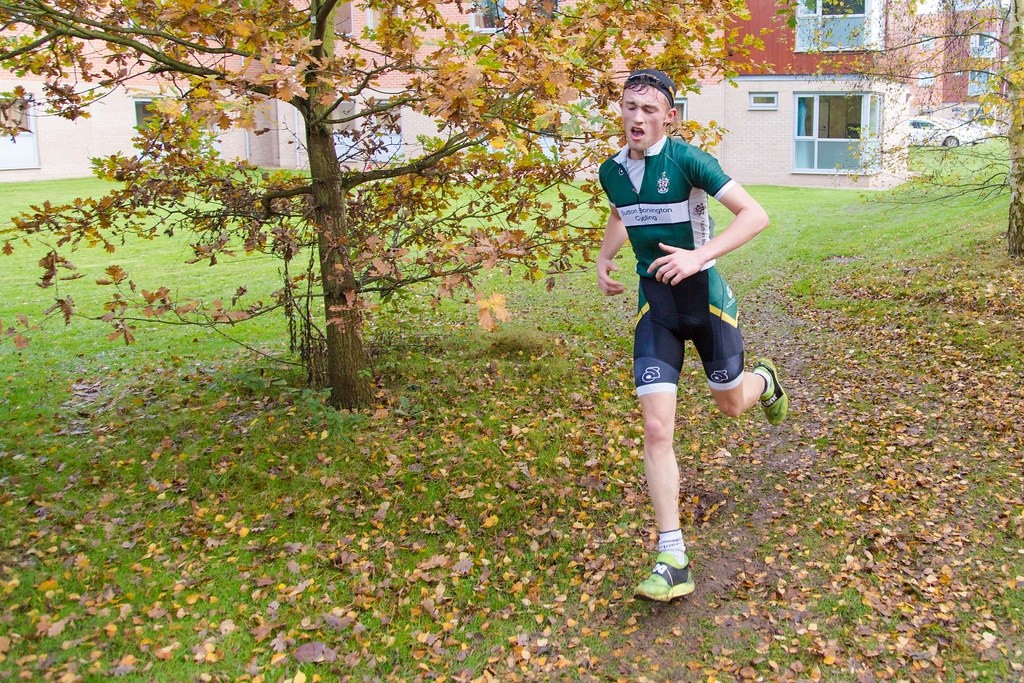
[628,68,678,98]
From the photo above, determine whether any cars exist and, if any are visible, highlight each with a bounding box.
[905,116,979,150]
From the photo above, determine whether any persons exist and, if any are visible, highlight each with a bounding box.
[594,71,791,601]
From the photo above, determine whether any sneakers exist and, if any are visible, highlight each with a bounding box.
[753,358,789,427]
[635,551,697,601]
